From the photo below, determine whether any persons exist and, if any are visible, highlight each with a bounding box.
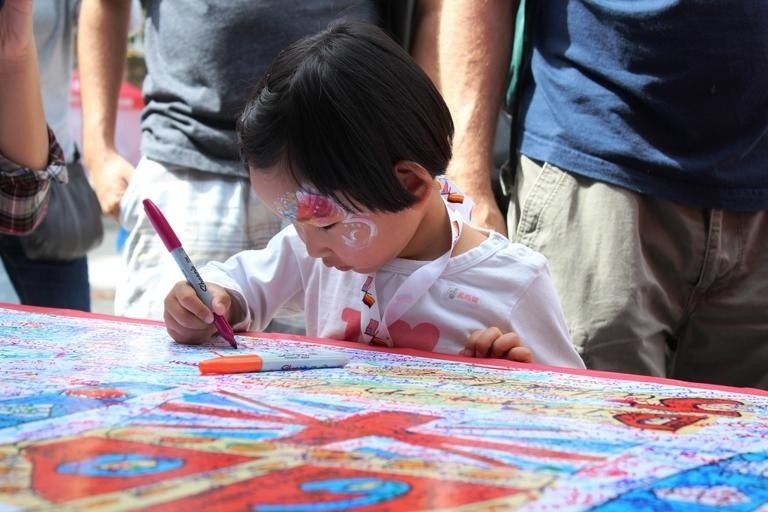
[163,17,587,379]
[73,0,422,336]
[0,0,90,312]
[0,0,68,236]
[411,0,768,387]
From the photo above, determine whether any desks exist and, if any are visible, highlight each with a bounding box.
[1,300,766,512]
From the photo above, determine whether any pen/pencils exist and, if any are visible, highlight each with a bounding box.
[198,353,350,374]
[145,198,238,348]
